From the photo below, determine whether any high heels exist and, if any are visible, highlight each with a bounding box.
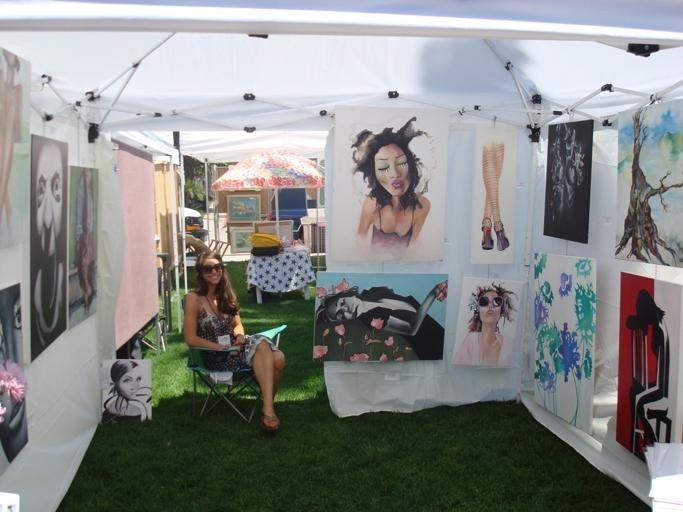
[481,216,494,250]
[495,221,510,251]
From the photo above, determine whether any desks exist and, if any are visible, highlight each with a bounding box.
[245,246,316,303]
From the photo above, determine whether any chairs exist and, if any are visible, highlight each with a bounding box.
[205,238,229,267]
[178,320,283,424]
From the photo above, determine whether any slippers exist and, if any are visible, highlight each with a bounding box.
[259,412,280,431]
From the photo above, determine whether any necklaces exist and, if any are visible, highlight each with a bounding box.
[206,297,222,320]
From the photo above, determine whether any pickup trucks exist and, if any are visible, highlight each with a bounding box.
[180,206,209,248]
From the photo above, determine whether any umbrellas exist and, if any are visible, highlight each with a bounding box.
[211,145,325,241]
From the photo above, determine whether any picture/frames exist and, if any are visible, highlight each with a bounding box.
[251,219,295,246]
[227,228,256,254]
[224,193,262,225]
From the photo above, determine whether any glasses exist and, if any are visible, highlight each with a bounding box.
[200,262,222,272]
[479,295,503,307]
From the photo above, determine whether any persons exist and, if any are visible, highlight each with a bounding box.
[32,142,68,349]
[0,49,24,228]
[0,284,34,465]
[351,117,431,260]
[102,359,151,423]
[76,168,96,313]
[460,283,518,364]
[316,281,449,361]
[183,250,285,432]
[469,139,519,252]
